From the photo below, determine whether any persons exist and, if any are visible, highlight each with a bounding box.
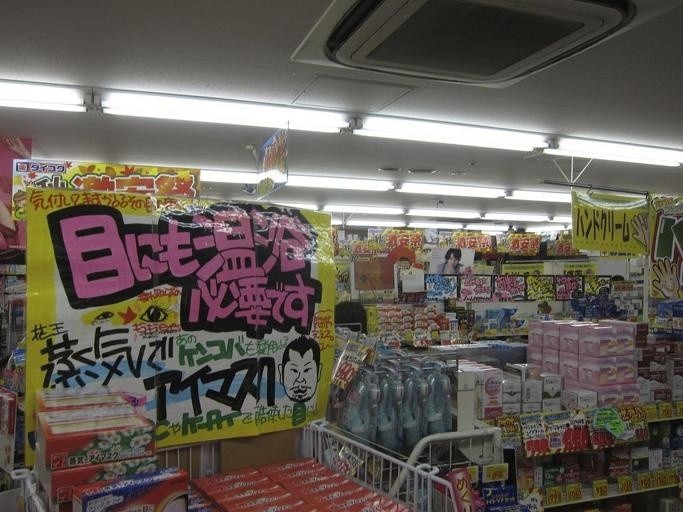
[275,334,324,410]
[437,248,464,274]
